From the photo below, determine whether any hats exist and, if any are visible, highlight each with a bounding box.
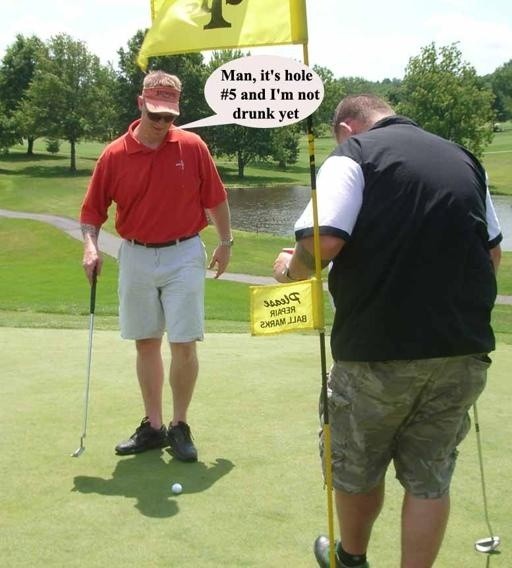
[142,85,181,116]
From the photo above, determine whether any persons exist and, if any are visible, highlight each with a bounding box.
[79,70,233,462]
[272,92,503,568]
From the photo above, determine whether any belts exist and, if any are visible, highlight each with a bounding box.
[125,234,199,247]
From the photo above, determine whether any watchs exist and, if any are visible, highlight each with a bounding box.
[282,264,293,282]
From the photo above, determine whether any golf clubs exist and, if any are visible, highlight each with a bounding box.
[72,266,97,457]
[473,402,499,554]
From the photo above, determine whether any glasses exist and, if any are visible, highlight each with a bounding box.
[144,103,173,123]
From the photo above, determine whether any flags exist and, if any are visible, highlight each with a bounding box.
[137,0,309,70]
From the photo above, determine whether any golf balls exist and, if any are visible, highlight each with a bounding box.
[172,483,183,494]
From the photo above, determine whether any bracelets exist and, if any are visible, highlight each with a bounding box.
[220,237,233,248]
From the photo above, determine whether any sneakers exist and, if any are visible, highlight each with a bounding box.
[315,536,366,568]
[115,418,168,455]
[168,422,197,460]
